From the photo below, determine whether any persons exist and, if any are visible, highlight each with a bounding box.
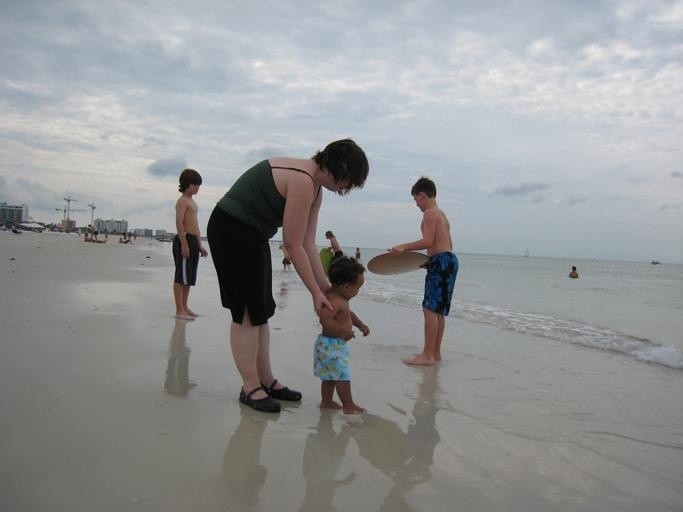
[279,242,291,271]
[171,169,207,321]
[206,137,370,413]
[387,174,458,365]
[0,221,137,245]
[311,256,369,414]
[567,265,579,278]
[325,230,342,261]
[354,247,360,260]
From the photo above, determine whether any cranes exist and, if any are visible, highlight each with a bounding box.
[56,195,97,233]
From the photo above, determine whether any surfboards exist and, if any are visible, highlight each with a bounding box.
[319,248,333,277]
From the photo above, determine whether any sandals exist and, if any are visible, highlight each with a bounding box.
[260,377,302,403]
[238,385,281,412]
